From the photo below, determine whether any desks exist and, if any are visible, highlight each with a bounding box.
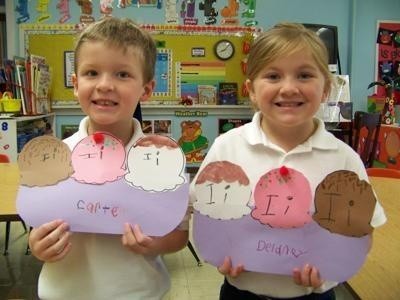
[1,162,206,268]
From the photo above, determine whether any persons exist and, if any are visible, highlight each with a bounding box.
[189,20,387,300]
[29,15,190,300]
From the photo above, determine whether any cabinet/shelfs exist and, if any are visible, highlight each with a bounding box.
[0,113,57,161]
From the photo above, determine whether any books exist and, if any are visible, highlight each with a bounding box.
[0,52,54,114]
[197,84,218,107]
[218,81,238,106]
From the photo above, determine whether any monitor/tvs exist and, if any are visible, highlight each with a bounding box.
[303,24,338,73]
